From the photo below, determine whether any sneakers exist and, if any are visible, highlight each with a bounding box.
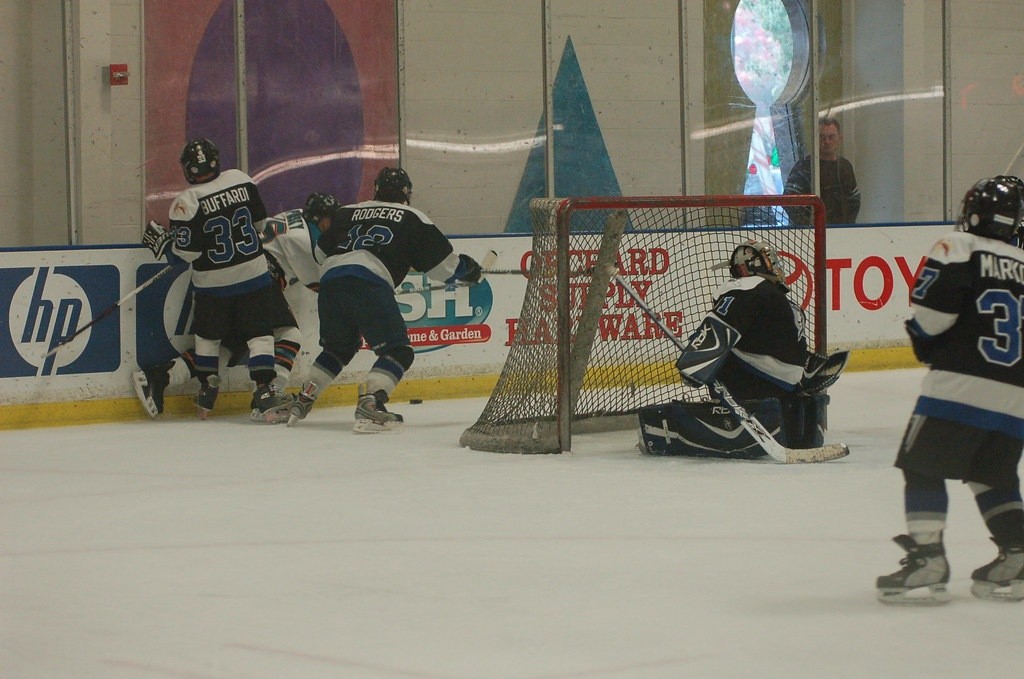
[970,538,1024,602]
[249,383,297,425]
[287,380,318,427]
[353,384,403,432]
[132,361,176,419]
[191,375,221,420]
[876,529,952,607]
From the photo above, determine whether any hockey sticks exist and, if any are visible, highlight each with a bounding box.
[409,398,423,405]
[609,262,850,465]
[39,262,173,360]
[395,249,497,295]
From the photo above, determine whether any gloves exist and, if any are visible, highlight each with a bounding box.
[142,220,174,259]
[457,254,482,282]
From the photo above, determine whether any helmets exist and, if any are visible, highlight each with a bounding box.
[961,175,1024,250]
[303,192,343,233]
[730,240,785,284]
[181,138,220,184]
[374,167,412,205]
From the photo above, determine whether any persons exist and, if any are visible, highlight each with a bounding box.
[781,116,862,229]
[875,176,1024,601]
[126,136,344,425]
[287,168,482,437]
[637,238,853,459]
